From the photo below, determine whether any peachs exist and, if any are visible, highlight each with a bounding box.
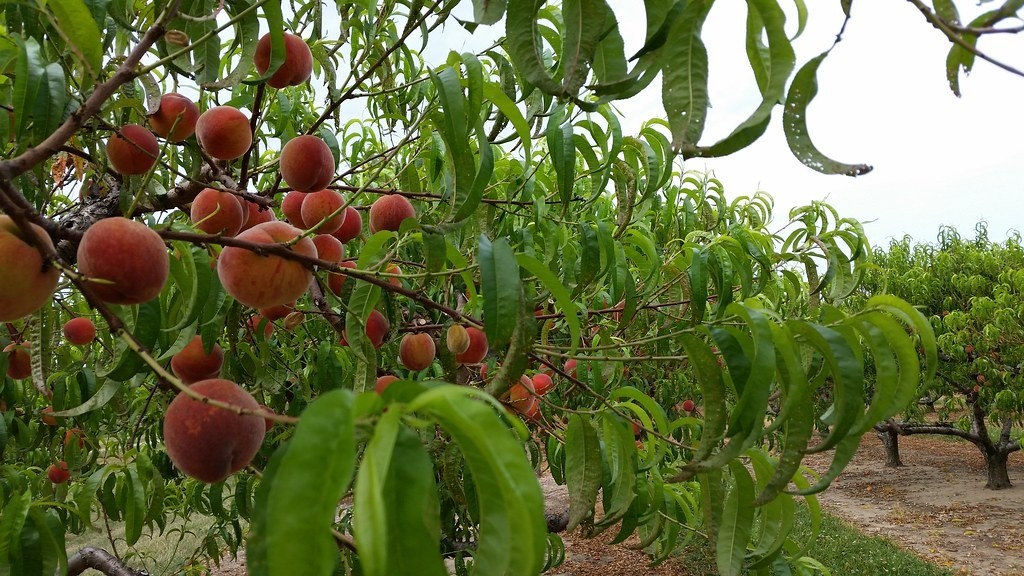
[0,27,1007,489]
